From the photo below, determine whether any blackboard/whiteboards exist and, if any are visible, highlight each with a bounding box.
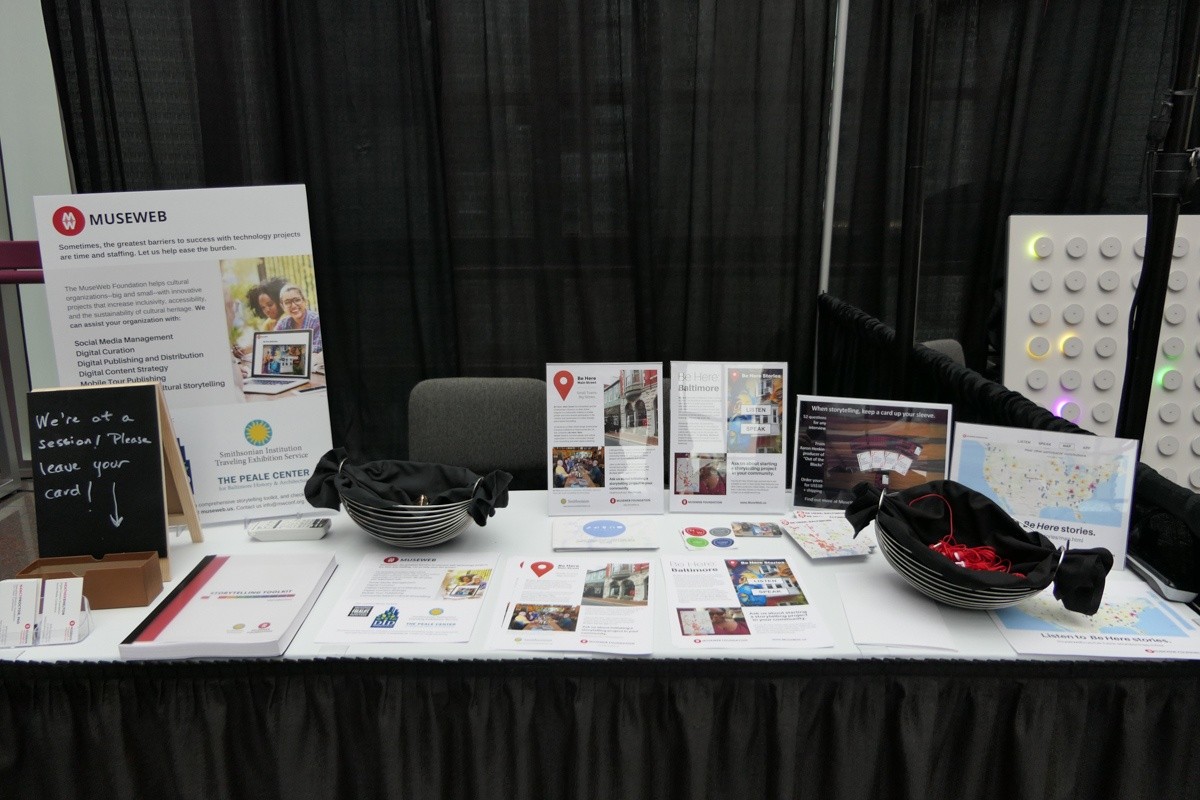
[25,381,205,583]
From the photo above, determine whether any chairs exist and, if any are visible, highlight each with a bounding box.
[407,377,549,490]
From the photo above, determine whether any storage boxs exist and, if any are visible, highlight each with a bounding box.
[16,549,164,613]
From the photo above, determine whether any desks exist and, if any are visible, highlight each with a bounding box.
[0,487,1199,800]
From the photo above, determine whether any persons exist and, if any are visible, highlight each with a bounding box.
[262,346,302,372]
[708,608,750,635]
[700,467,723,494]
[513,607,573,630]
[273,284,323,352]
[468,575,487,589]
[461,574,472,583]
[554,454,602,484]
[232,278,286,358]
